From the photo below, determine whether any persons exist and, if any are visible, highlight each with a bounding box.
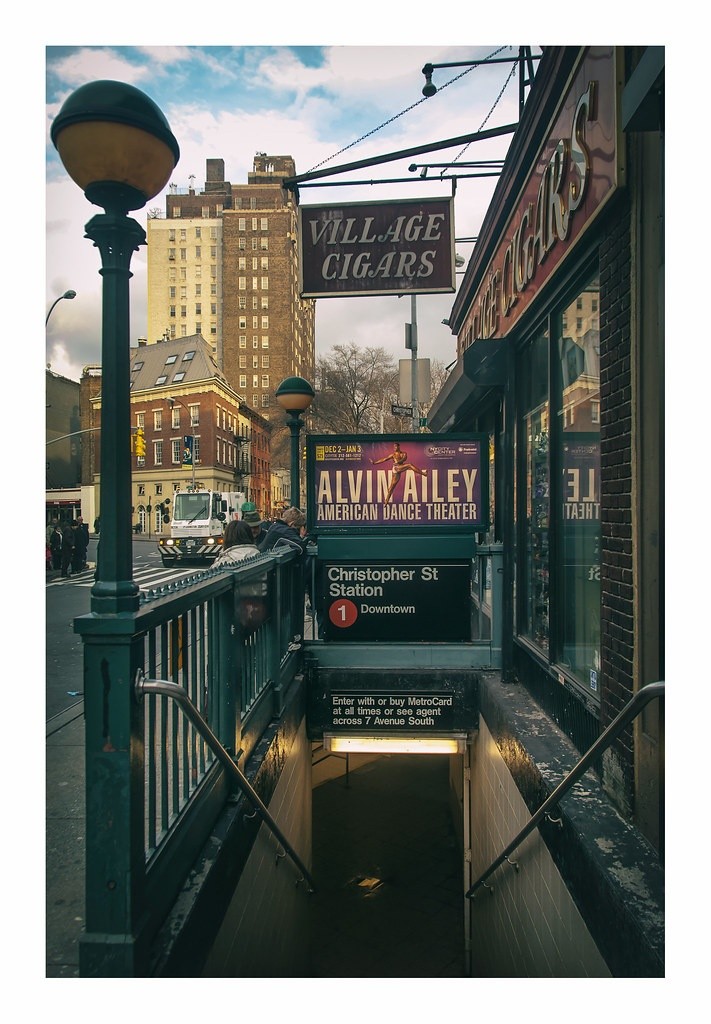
[369,443,428,508]
[257,505,316,674]
[61,520,78,578]
[204,519,261,720]
[46,517,58,570]
[50,526,63,570]
[77,517,89,573]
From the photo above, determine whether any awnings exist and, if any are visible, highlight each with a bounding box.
[427,338,508,433]
[523,337,584,414]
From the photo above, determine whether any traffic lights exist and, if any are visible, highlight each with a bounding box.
[136,436,146,457]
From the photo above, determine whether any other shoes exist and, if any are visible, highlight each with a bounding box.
[61,574,71,578]
[70,570,79,574]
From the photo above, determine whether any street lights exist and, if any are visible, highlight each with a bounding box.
[411,252,466,433]
[276,376,315,511]
[50,79,182,616]
[165,396,197,490]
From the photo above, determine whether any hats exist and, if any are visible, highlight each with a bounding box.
[242,511,264,527]
[70,520,78,526]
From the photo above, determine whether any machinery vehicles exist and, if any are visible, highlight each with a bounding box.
[158,488,247,568]
